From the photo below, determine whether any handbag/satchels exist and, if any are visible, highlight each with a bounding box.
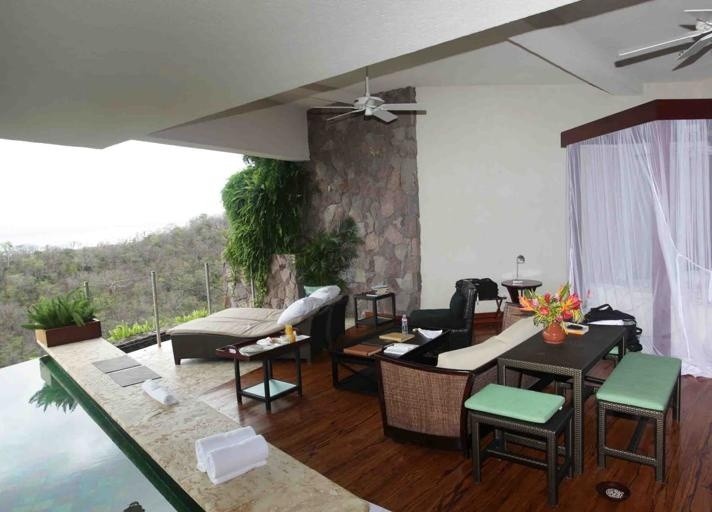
[457,278,498,300]
[584,304,642,352]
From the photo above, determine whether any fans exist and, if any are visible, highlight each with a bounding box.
[618,9,712,69]
[311,75,425,123]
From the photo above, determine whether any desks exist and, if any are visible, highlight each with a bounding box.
[215,327,310,413]
[497,323,627,474]
[502,278,542,302]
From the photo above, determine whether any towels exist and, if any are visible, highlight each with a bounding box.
[194,426,256,472]
[206,435,268,485]
[143,379,179,405]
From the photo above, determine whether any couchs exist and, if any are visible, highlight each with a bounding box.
[374,301,553,460]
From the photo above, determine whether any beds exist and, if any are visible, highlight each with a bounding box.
[166,294,349,366]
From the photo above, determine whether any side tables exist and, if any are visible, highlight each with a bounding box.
[353,290,396,331]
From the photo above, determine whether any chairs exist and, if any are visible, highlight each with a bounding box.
[409,280,476,348]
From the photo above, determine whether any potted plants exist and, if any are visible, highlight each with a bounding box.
[20,286,101,348]
[284,216,364,296]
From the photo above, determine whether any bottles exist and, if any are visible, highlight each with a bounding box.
[401,315,408,336]
[285,324,297,342]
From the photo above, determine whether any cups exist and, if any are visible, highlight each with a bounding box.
[280,335,292,345]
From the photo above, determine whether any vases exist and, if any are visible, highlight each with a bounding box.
[543,318,568,344]
[543,321,565,344]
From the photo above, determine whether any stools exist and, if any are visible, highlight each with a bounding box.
[606,347,628,366]
[465,383,574,503]
[595,351,681,482]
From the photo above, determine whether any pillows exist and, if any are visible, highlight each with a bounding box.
[310,285,340,300]
[278,297,320,326]
[309,285,341,302]
[278,296,322,324]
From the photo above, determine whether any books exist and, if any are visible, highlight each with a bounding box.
[343,332,415,357]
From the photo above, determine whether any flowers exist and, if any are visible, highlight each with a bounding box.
[517,281,585,335]
